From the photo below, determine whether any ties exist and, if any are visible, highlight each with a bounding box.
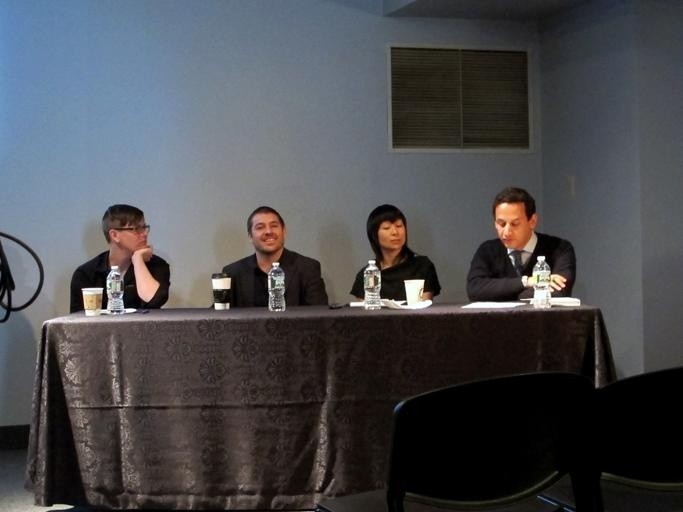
[510,250,523,275]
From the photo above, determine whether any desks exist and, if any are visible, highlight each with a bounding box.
[23,298,622,512]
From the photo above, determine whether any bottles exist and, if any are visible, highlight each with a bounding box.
[362,260,382,311]
[104,264,125,317]
[531,254,553,309]
[266,262,287,313]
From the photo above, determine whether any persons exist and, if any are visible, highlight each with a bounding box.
[211,204,328,307]
[67,202,170,316]
[464,182,575,302]
[348,201,441,303]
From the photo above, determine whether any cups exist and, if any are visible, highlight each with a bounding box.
[80,287,103,317]
[210,272,233,312]
[403,278,426,308]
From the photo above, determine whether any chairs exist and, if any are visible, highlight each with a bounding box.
[308,368,604,508]
[590,363,682,509]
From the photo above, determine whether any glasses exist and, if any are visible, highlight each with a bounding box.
[113,225,151,234]
